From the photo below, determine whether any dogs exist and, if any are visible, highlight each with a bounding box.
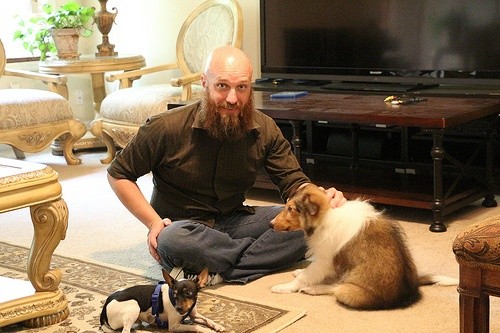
[268,183,459,311]
[99,266,225,333]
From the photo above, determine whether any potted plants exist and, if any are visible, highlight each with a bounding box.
[16,1,99,61]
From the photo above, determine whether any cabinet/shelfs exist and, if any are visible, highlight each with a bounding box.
[245,92,500,232]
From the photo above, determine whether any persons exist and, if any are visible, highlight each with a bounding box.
[107,46,347,285]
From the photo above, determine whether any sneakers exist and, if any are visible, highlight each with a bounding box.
[168,267,224,290]
[296,253,311,263]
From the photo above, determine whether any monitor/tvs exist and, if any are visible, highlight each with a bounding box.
[259,0,500,92]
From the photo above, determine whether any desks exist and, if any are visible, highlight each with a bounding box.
[42,56,146,156]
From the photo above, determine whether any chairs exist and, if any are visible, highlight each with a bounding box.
[0,39,86,166]
[89,1,244,164]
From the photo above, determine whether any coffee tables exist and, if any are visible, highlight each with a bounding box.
[0,156,71,330]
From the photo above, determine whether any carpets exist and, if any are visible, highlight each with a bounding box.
[0,241,308,333]
